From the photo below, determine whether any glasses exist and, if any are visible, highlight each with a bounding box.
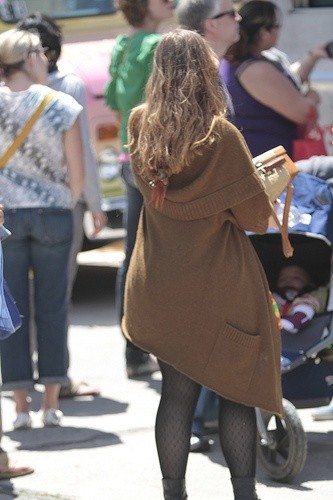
[214,9,239,20]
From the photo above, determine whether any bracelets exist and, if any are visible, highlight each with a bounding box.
[308,51,318,60]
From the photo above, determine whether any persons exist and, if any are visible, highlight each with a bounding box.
[121,30,285,500]
[0,0,333,481]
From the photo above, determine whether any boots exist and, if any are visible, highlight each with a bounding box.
[231,477,258,500]
[162,479,187,500]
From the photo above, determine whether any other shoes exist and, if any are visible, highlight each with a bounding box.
[14,413,32,430]
[42,409,63,427]
[0,453,33,478]
[59,379,101,399]
[126,357,161,377]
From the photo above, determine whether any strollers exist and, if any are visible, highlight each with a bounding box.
[240,173,333,484]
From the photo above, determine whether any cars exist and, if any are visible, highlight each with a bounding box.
[0,0,251,290]
[265,0,333,128]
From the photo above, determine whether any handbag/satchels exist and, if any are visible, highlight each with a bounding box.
[252,145,300,258]
[293,105,327,163]
